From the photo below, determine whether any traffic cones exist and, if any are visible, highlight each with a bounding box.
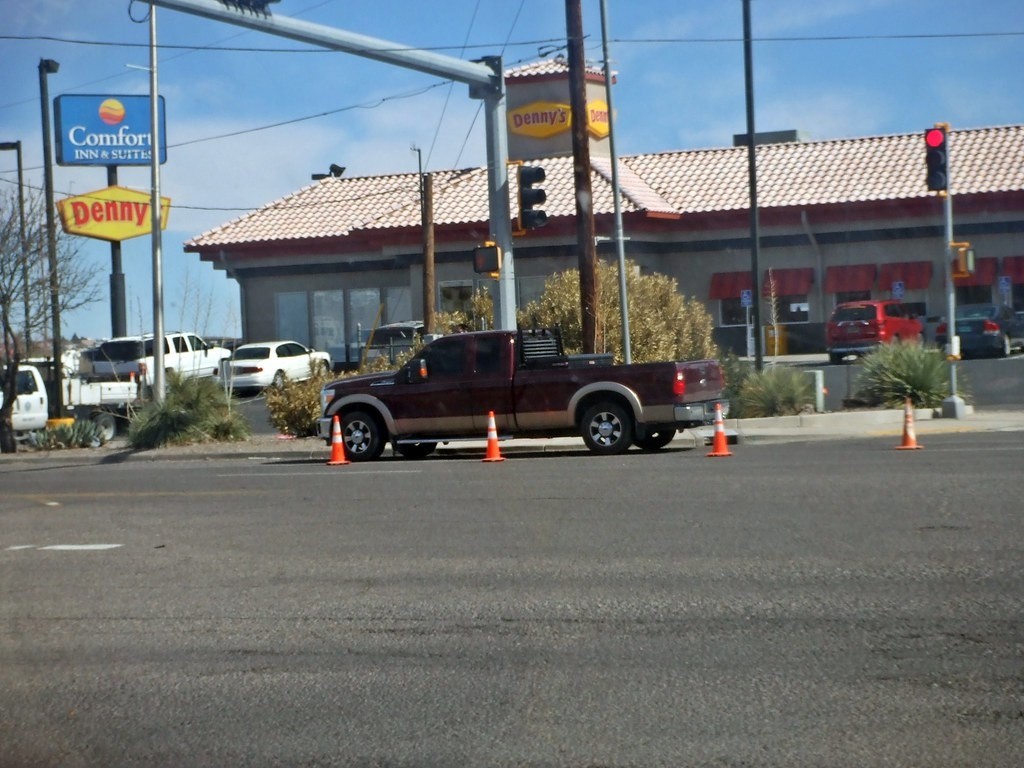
[704,403,732,457]
[325,413,351,466]
[480,411,506,463]
[892,398,925,451]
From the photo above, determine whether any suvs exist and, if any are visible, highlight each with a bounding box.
[825,301,927,367]
[93,330,232,388]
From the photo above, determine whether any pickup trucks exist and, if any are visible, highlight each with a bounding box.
[318,322,731,463]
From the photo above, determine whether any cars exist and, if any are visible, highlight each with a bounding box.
[935,302,1024,358]
[218,340,331,398]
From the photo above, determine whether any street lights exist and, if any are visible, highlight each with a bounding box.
[0,139,31,359]
[36,56,61,417]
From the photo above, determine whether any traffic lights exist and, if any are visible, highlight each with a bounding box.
[959,247,976,275]
[923,128,948,191]
[472,246,502,275]
[516,164,548,230]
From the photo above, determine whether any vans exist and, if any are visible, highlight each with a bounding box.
[0,365,49,432]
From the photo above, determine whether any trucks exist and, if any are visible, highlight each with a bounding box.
[18,357,137,443]
